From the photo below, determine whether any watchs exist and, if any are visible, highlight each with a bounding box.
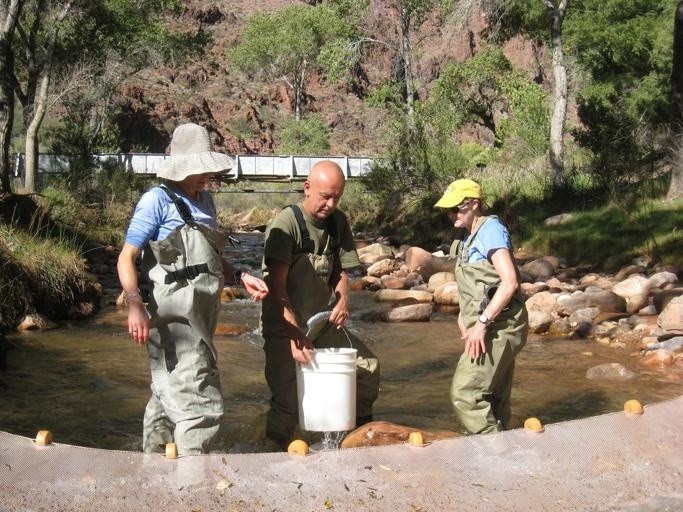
[476,314,492,328]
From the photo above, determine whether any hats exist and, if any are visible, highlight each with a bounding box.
[156,123,232,181]
[433,178,481,208]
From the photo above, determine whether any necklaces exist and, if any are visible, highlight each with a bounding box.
[124,291,145,306]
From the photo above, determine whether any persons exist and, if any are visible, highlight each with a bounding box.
[432,176,529,434]
[118,123,268,456]
[263,160,380,451]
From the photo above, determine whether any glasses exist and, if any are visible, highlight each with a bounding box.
[451,202,470,214]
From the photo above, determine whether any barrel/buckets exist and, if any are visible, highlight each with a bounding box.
[294,311,356,432]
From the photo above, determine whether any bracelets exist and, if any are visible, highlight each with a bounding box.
[233,266,253,286]
